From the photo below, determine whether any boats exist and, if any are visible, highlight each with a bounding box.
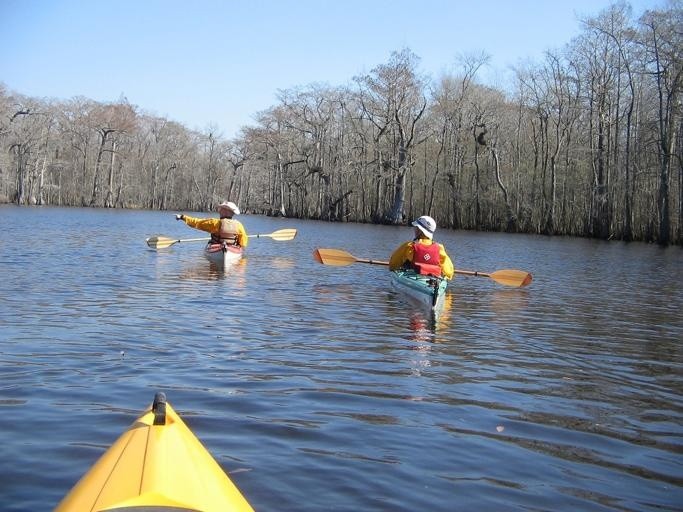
[204,240,245,267]
[390,264,451,308]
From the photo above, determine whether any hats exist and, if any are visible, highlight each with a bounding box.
[411,215,436,238]
[214,200,241,215]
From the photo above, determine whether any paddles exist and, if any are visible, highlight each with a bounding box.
[146,229,297,249]
[314,249,532,288]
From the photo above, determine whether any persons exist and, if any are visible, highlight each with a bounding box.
[173,202,248,247]
[389,215,455,280]
